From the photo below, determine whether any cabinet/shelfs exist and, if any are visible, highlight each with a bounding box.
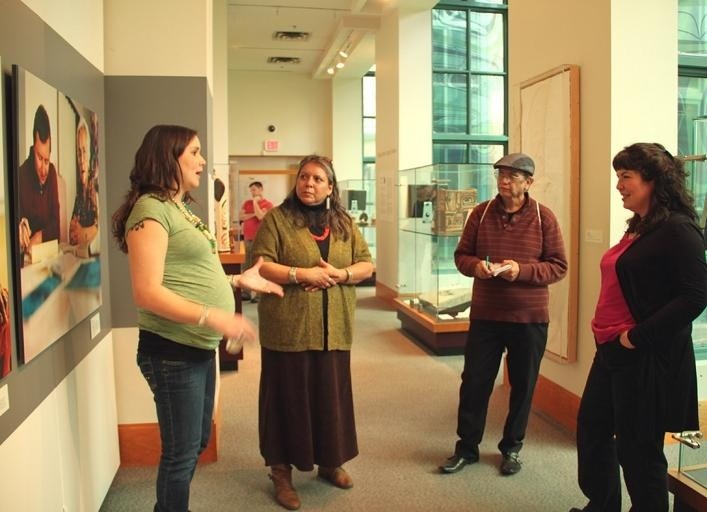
[338,180,377,288]
[666,117,707,511]
[393,163,504,357]
[214,161,244,373]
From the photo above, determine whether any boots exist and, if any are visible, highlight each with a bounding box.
[318,465,353,488]
[272,466,300,510]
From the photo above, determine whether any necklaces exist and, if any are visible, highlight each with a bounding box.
[305,220,330,242]
[169,196,218,255]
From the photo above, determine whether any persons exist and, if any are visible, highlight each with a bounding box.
[237,179,275,306]
[66,117,99,259]
[17,102,62,266]
[566,139,705,512]
[248,151,376,510]
[109,124,286,510]
[437,152,570,478]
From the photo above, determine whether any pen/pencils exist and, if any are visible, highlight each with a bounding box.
[486,256,489,269]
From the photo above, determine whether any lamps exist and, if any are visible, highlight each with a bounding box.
[325,41,351,77]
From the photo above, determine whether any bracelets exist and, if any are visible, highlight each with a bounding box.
[195,304,208,328]
[288,266,299,286]
[342,266,352,286]
[226,273,233,286]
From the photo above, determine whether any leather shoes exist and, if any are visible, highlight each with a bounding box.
[500,452,521,474]
[441,454,479,473]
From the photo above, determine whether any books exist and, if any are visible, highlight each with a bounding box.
[489,261,512,279]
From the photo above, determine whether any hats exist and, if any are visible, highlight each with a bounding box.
[494,153,535,175]
